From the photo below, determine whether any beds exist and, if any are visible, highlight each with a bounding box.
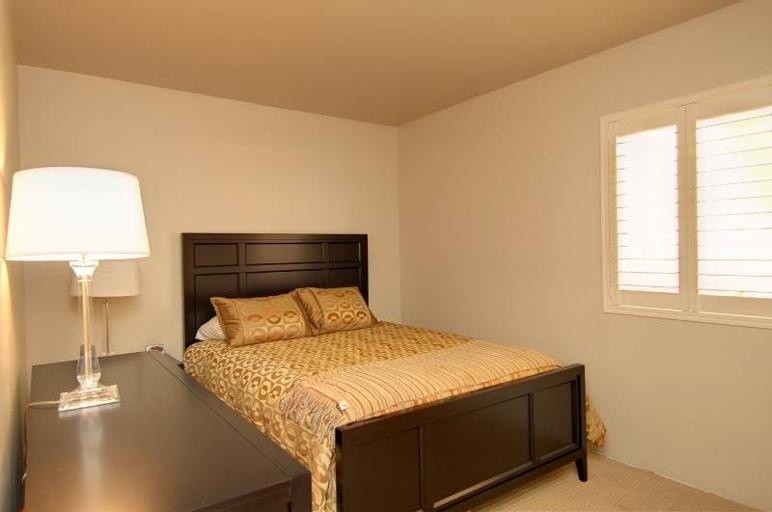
[177,227,598,511]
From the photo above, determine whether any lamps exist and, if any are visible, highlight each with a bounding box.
[64,256,143,359]
[4,165,155,418]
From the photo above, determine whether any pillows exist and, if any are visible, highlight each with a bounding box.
[294,280,379,335]
[194,314,226,343]
[208,288,314,350]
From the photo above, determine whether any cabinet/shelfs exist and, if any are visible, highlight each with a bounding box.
[22,341,314,511]
